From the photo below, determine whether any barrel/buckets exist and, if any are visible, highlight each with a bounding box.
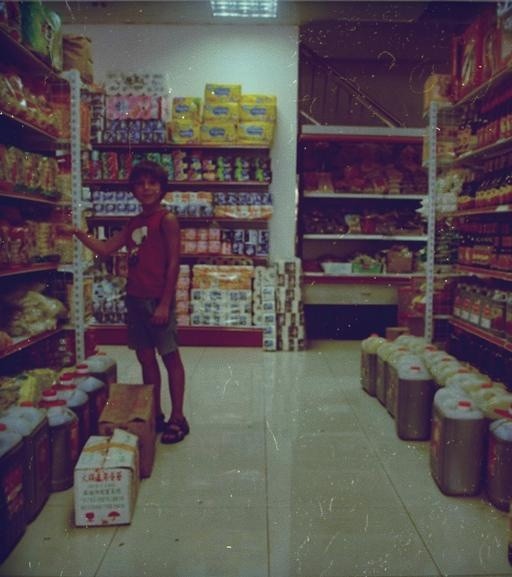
[0,350,117,565]
[359,282,512,514]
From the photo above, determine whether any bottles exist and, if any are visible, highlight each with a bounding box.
[450,86,511,272]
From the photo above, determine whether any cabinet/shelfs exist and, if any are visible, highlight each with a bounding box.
[297,123,428,339]
[2,30,273,365]
[426,63,510,364]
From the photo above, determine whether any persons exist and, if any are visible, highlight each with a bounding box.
[53,160,189,444]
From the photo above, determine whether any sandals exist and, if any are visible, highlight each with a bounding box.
[156,413,190,444]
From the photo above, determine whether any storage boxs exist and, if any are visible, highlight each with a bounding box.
[388,250,413,272]
[352,262,383,273]
[73,382,159,526]
[321,262,353,273]
